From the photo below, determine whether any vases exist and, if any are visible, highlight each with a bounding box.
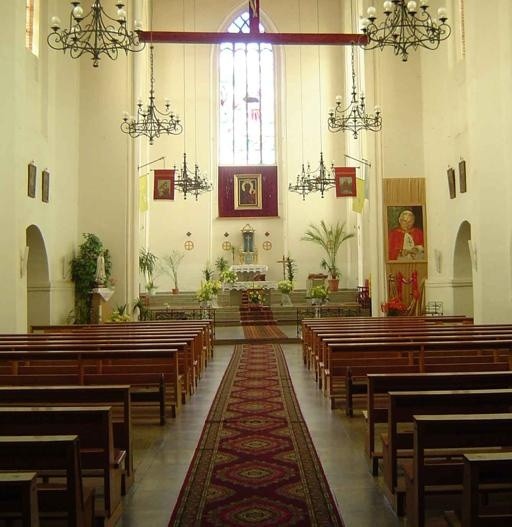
[280,294,294,306]
[312,298,323,305]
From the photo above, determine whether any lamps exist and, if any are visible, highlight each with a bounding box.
[288,0,337,201]
[46,1,148,69]
[174,0,215,201]
[328,1,384,139]
[120,1,183,146]
[357,0,452,64]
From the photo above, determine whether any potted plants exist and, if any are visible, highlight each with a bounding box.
[301,220,358,291]
[139,248,186,295]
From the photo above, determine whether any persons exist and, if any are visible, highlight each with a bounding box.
[389,209,425,260]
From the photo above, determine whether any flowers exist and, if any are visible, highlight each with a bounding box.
[277,280,295,293]
[306,285,327,298]
[197,270,239,308]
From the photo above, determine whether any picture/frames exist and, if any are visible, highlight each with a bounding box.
[384,202,428,264]
[233,174,263,211]
